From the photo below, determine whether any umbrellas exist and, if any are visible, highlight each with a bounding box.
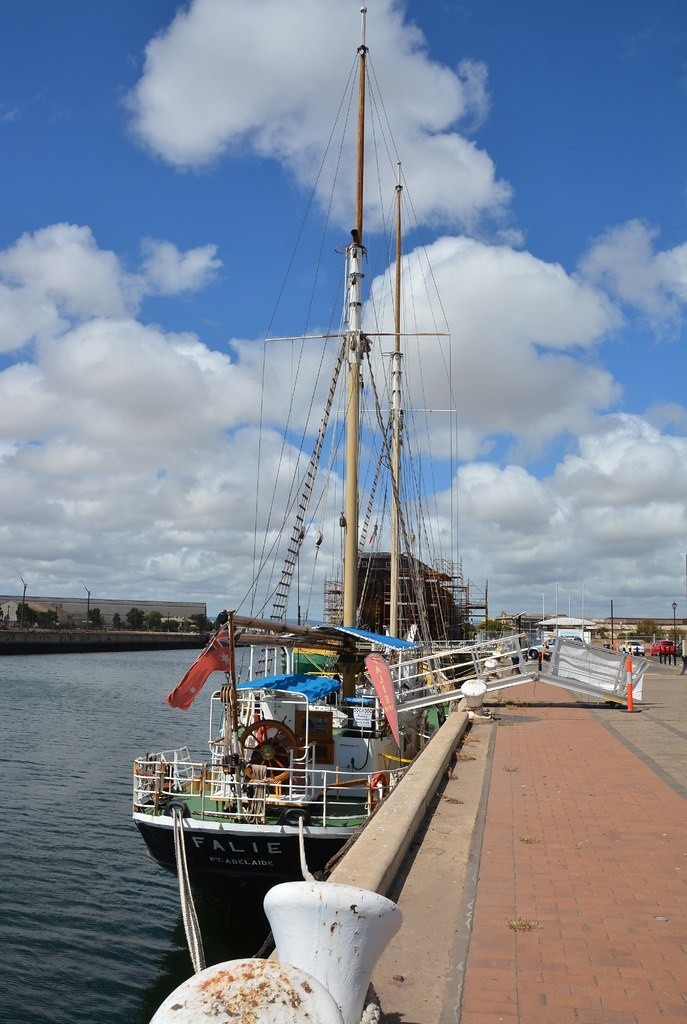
[532,617,597,625]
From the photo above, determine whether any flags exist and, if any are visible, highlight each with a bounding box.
[162,620,231,711]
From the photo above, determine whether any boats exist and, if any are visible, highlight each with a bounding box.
[133,5,490,928]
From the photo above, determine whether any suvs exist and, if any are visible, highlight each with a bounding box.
[528,637,586,660]
[618,640,646,656]
[650,640,675,657]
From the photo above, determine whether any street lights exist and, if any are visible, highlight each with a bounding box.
[672,600,677,666]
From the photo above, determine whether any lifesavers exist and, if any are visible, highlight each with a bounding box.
[284,809,308,826]
[366,773,389,811]
[167,800,186,818]
